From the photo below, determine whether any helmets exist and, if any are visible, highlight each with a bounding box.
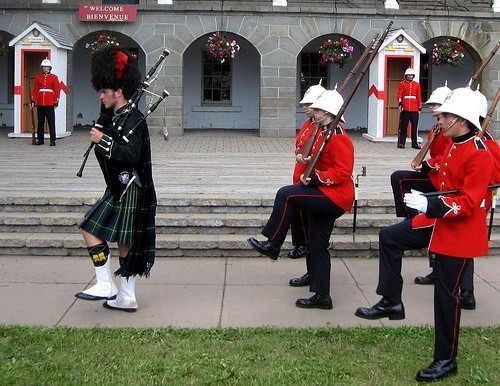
[299,78,326,104]
[309,82,346,122]
[40,59,52,66]
[404,67,416,80]
[426,77,489,131]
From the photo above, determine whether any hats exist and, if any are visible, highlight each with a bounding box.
[92,46,140,99]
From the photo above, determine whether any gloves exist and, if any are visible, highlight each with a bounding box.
[404,188,428,213]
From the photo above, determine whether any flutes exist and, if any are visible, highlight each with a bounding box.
[76,48,171,179]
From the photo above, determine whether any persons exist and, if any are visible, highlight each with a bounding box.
[76,45,157,312]
[246,82,355,309]
[288,79,328,286]
[390,81,500,310]
[356,77,493,382]
[30,56,60,146]
[397,67,422,150]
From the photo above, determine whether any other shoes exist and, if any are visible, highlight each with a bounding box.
[411,144,421,149]
[49,141,56,146]
[398,143,405,148]
[35,140,44,145]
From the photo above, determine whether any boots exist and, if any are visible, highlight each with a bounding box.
[103,276,138,311]
[74,251,118,301]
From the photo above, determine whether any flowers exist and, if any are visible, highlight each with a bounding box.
[86,32,119,52]
[207,32,240,61]
[320,37,353,66]
[431,40,465,68]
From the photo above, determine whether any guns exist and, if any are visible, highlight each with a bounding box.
[294,20,394,184]
[28,81,36,145]
[412,41,500,170]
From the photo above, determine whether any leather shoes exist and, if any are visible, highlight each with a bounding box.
[290,271,310,286]
[415,358,458,382]
[286,246,305,258]
[415,271,435,285]
[354,299,405,320]
[458,290,476,310]
[295,293,333,311]
[247,237,280,260]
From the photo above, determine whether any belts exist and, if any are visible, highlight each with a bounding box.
[479,199,486,207]
[404,96,417,99]
[38,88,53,92]
